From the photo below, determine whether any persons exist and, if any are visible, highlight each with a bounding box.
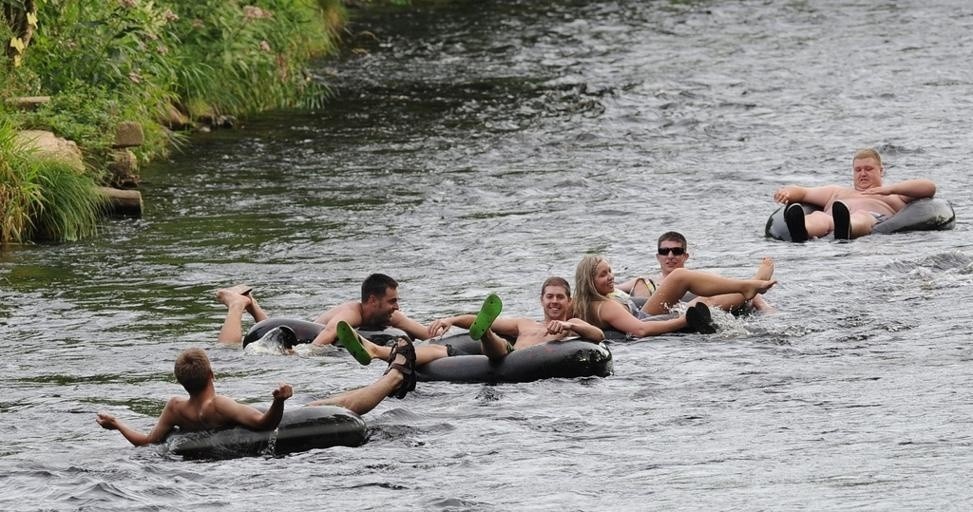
[610,232,773,312]
[92,337,416,449]
[560,253,780,340]
[213,272,440,356]
[772,149,936,245]
[334,277,605,368]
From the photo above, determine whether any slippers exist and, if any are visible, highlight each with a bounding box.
[784,202,808,243]
[831,201,852,240]
[336,319,371,366]
[685,301,716,334]
[468,294,503,341]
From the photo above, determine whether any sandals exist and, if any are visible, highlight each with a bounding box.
[385,335,416,400]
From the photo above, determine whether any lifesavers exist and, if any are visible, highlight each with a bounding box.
[573,290,753,342]
[766,196,955,244]
[414,330,614,385]
[243,315,413,350]
[168,403,367,461]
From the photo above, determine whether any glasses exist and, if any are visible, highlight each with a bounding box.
[657,246,686,255]
